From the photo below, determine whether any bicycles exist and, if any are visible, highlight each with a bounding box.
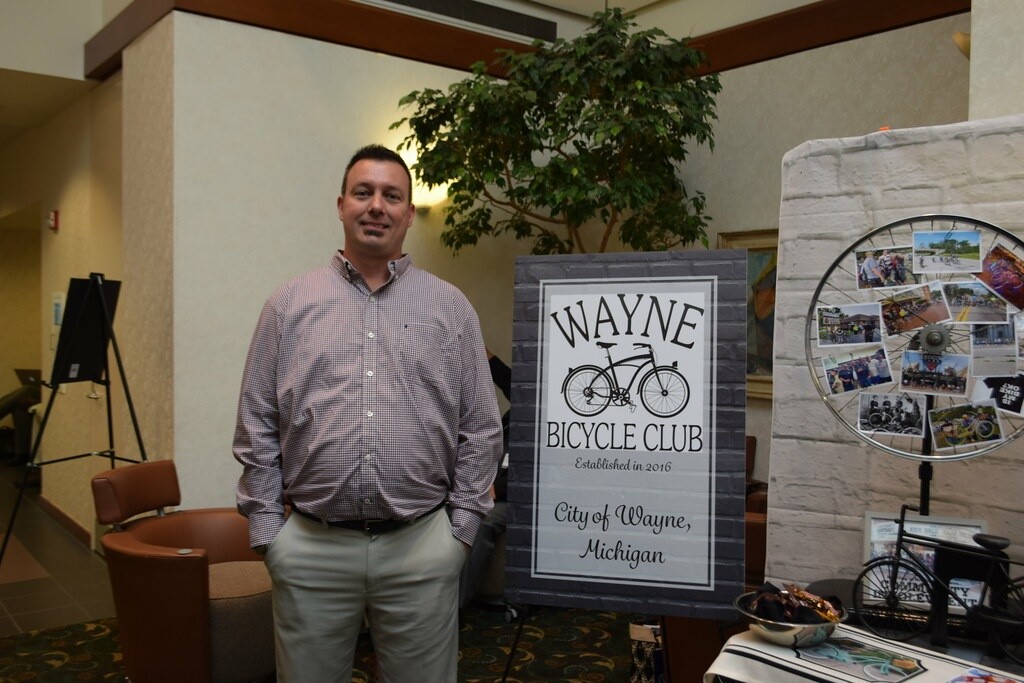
[852,501,1024,666]
[560,341,690,418]
[945,411,996,446]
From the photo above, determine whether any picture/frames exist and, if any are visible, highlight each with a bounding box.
[716,228,779,399]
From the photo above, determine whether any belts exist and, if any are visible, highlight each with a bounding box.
[291,497,447,535]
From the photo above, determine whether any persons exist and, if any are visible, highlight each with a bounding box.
[940,409,992,442]
[860,251,909,284]
[229,146,505,683]
[869,394,922,431]
[829,324,843,342]
[853,322,863,335]
[919,255,924,268]
[829,353,891,392]
[884,302,933,331]
[951,293,998,307]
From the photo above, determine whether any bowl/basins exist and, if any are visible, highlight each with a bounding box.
[734,591,848,646]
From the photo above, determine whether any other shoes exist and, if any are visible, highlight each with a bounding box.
[459,615,465,627]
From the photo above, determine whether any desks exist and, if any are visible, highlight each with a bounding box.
[702,579,1024,683]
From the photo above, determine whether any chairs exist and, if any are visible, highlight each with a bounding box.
[91,460,276,683]
